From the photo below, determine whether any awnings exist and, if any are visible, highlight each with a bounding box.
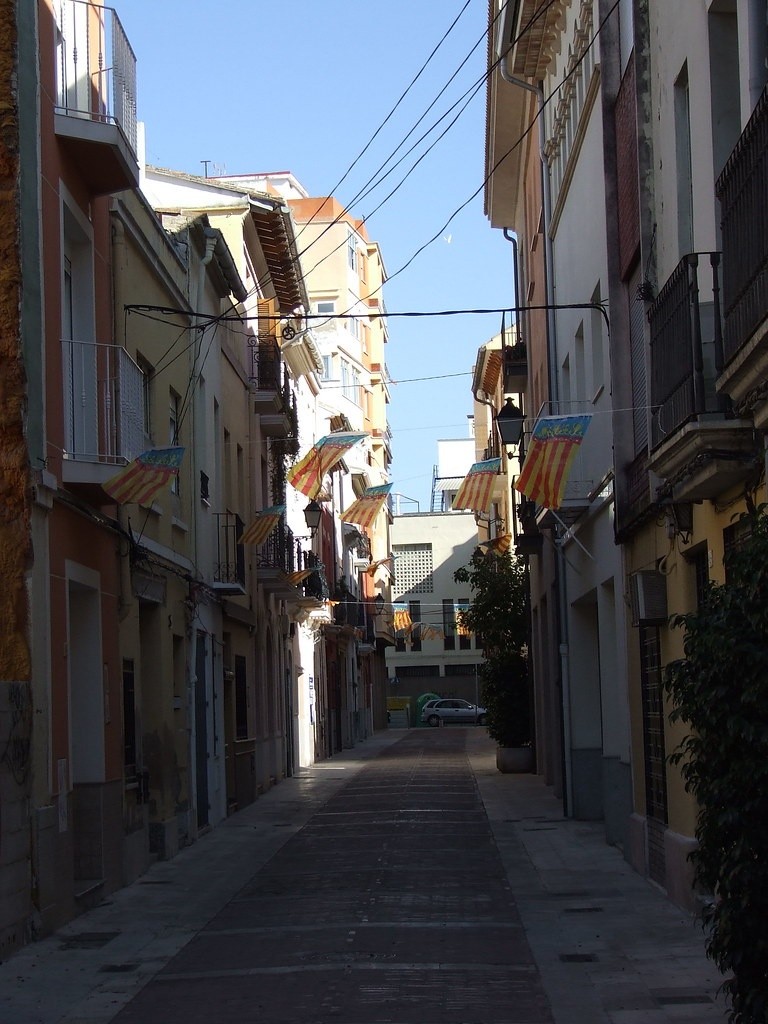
[432,478,466,491]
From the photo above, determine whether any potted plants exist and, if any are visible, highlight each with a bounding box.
[449,546,534,773]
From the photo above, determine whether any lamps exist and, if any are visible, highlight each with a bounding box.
[471,547,486,571]
[294,500,323,540]
[368,593,385,616]
[493,397,532,445]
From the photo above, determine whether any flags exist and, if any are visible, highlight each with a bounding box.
[285,432,370,502]
[453,605,477,636]
[100,448,185,507]
[512,413,594,510]
[286,567,319,587]
[494,534,512,558]
[338,483,393,528]
[392,603,412,633]
[237,506,287,546]
[452,458,503,512]
[405,620,447,641]
[366,557,391,577]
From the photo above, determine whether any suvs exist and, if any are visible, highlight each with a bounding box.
[420,698,487,727]
